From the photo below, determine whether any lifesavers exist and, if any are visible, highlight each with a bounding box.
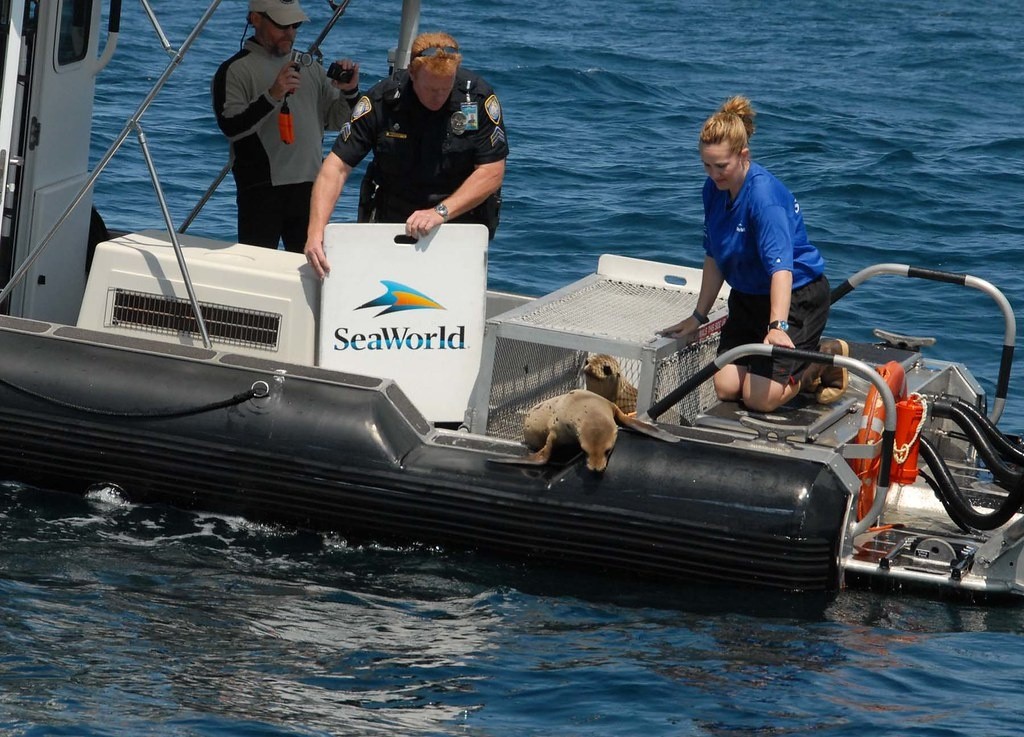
[844,358,911,526]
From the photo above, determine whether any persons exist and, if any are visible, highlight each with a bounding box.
[303,33,509,278]
[656,92,849,411]
[210,1,362,253]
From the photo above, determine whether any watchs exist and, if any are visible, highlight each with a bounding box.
[767,320,790,333]
[434,204,449,222]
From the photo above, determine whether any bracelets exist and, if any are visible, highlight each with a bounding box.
[693,309,710,324]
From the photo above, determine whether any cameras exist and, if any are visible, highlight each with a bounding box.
[327,63,355,84]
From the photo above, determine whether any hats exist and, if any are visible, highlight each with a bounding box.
[248,0,311,25]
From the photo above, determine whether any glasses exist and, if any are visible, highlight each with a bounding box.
[257,11,302,29]
[411,46,458,62]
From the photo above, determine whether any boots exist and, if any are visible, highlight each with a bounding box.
[799,338,849,405]
